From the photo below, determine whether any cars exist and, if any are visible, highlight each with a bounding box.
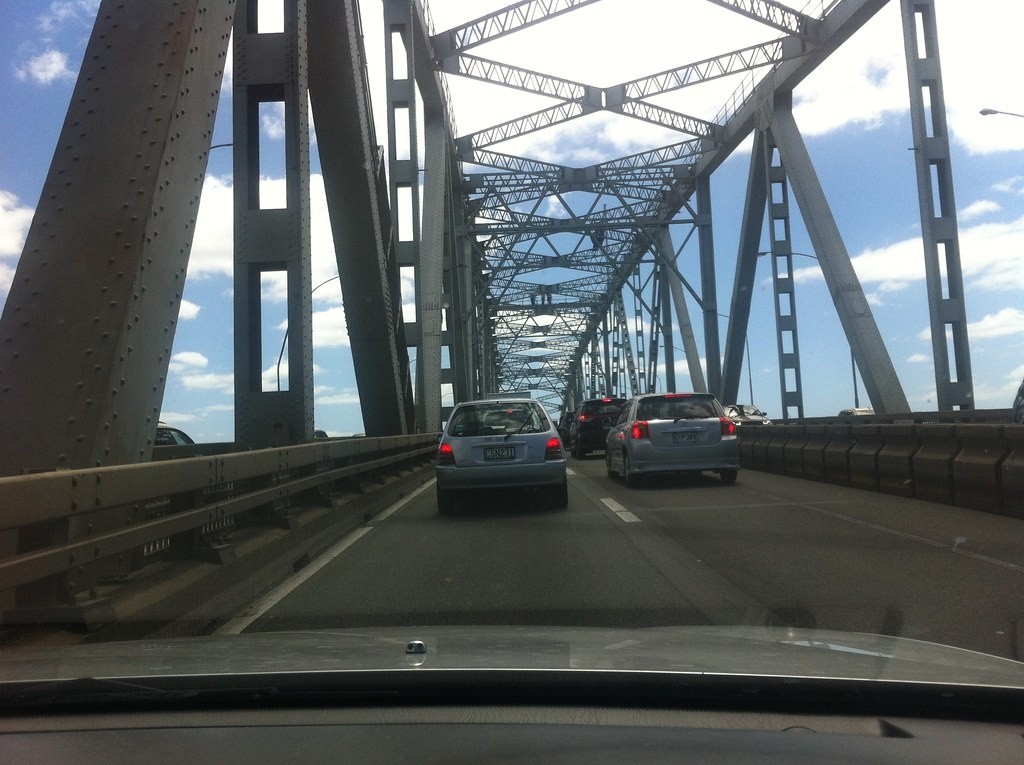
[313,427,329,439]
[837,407,875,415]
[600,392,742,489]
[720,402,774,427]
[156,419,197,445]
[558,411,592,453]
[566,399,627,459]
[436,398,567,507]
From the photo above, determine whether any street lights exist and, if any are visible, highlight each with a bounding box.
[755,251,861,408]
